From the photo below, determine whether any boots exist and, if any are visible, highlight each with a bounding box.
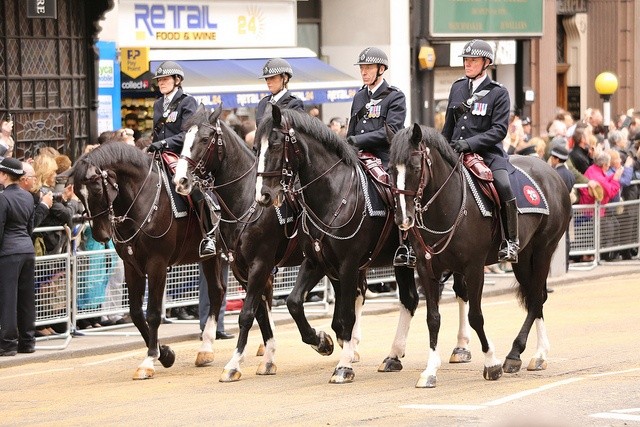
[396,239,417,269]
[498,197,519,263]
[193,199,217,255]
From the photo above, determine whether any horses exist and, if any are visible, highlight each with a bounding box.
[384,120,573,389]
[251,101,472,384]
[64,139,275,381]
[170,102,368,383]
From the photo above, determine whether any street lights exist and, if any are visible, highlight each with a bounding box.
[595,72,618,148]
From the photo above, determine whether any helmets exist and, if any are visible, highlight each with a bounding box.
[458,39,494,65]
[152,60,184,81]
[353,47,389,70]
[259,59,293,79]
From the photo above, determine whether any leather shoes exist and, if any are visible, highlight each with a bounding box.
[216,330,235,340]
[34,328,52,337]
[47,326,59,336]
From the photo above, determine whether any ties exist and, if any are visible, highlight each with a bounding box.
[367,91,373,99]
[163,98,170,112]
[470,82,472,96]
[270,99,276,105]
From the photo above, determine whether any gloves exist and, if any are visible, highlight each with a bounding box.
[451,139,470,154]
[146,142,164,154]
[346,136,357,147]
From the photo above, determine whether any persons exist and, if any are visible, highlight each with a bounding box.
[441,39,519,264]
[308,105,320,120]
[502,107,640,263]
[341,119,349,137]
[245,130,256,150]
[134,131,144,150]
[145,129,155,146]
[0,158,35,355]
[221,109,235,126]
[434,100,451,131]
[235,107,249,122]
[331,118,341,137]
[225,115,242,134]
[36,132,116,337]
[125,113,143,140]
[249,107,258,118]
[345,47,417,267]
[117,128,135,146]
[0,115,15,160]
[241,119,255,139]
[145,61,222,261]
[256,58,305,156]
[18,158,36,192]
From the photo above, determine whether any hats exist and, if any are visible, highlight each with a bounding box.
[0,157,26,176]
[550,145,569,161]
[516,145,539,158]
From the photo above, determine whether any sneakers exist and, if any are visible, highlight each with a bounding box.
[19,343,34,354]
[0,347,18,356]
[176,309,195,320]
